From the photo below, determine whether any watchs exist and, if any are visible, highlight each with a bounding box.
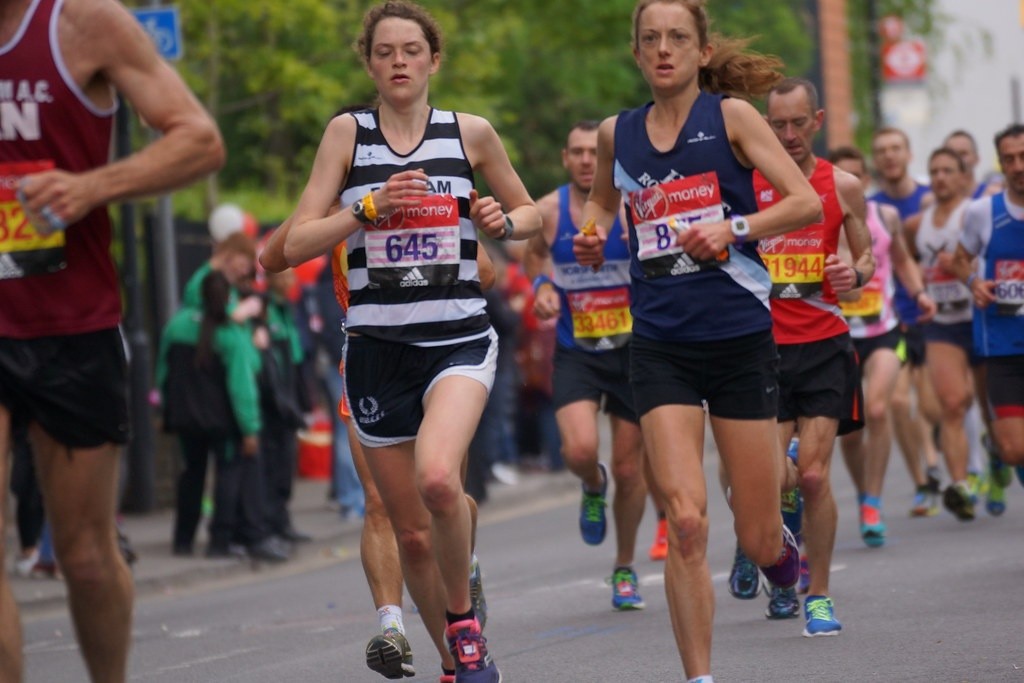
[351,198,373,224]
[494,214,514,242]
[730,214,750,249]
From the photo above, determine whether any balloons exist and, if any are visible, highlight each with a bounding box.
[208,203,257,244]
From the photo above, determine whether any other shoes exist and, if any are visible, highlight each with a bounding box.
[246,537,289,560]
[651,520,668,557]
[862,521,884,548]
[911,492,938,516]
[800,559,810,592]
[985,465,1005,515]
[943,480,974,519]
[279,526,311,542]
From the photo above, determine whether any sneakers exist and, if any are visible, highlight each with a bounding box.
[366,628,415,679]
[580,461,607,545]
[605,567,644,609]
[781,438,800,514]
[728,541,762,599]
[765,587,800,618]
[727,486,800,590]
[444,615,501,683]
[803,594,841,638]
[470,555,486,634]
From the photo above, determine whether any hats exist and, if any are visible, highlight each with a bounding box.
[208,204,243,243]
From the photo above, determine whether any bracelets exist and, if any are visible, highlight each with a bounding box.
[531,274,549,295]
[967,272,976,289]
[850,266,862,289]
[362,192,377,220]
[913,290,926,307]
[536,282,554,293]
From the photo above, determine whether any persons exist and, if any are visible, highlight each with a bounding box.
[525,0,876,683]
[827,124,1024,548]
[8,428,65,581]
[260,0,543,683]
[0,0,225,683]
[148,226,554,563]
